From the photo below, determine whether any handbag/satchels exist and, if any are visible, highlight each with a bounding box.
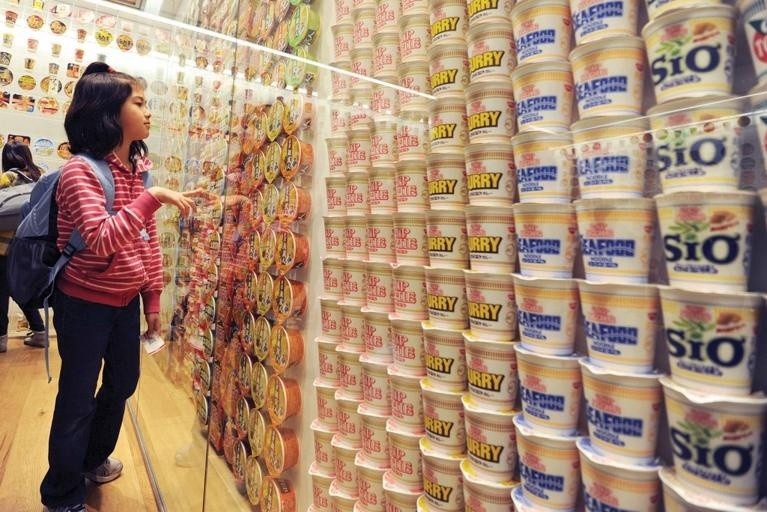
[1,168,35,232]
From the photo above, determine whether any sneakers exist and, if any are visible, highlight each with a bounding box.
[1,335,8,352]
[24,331,48,347]
[42,503,90,512]
[82,458,123,483]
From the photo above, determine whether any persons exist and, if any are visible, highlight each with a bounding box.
[39,61,201,512]
[0,140,52,353]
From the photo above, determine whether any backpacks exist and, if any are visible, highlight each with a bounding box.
[7,154,115,311]
